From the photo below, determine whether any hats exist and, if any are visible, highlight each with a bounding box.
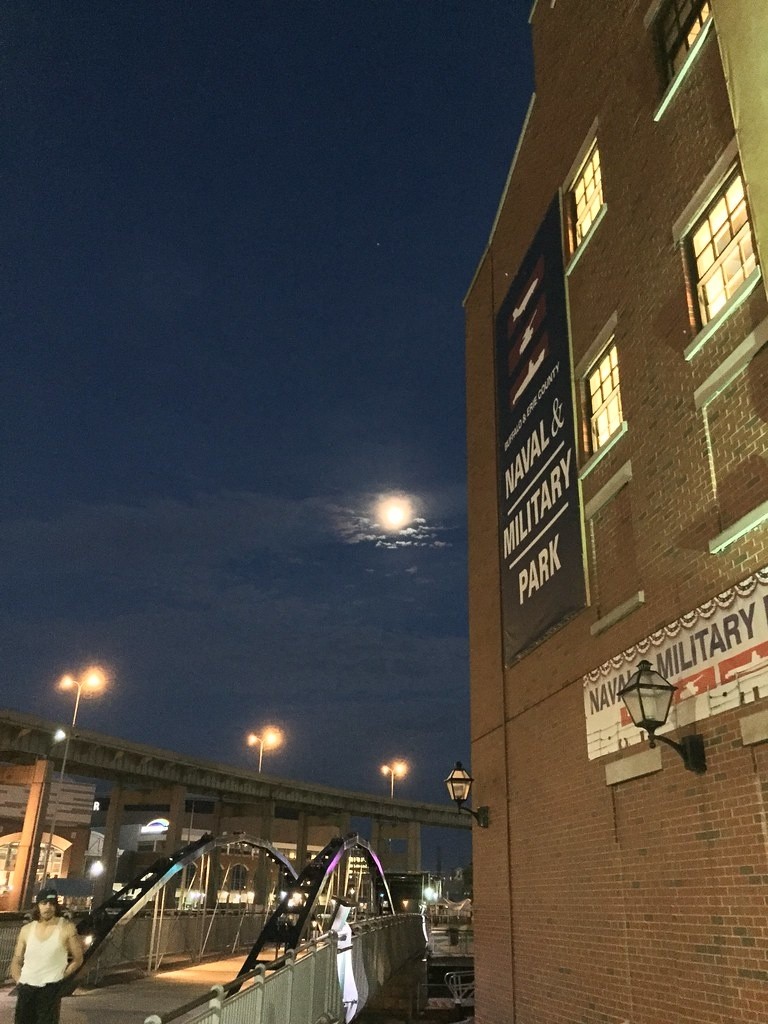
[36,887,58,902]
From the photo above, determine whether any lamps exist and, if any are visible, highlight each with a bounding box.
[616,661,706,778]
[443,762,488,829]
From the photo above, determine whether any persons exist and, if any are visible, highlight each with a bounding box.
[10,888,84,1024]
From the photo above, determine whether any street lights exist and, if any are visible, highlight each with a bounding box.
[40,670,102,890]
[246,728,280,772]
[379,762,405,798]
